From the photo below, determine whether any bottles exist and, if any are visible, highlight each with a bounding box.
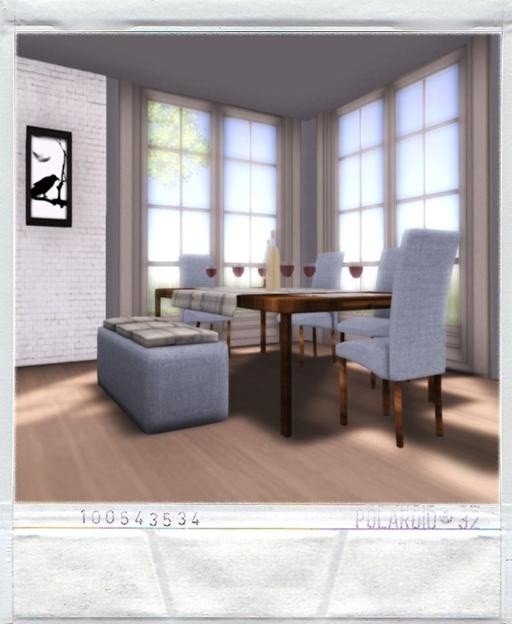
[265,239,281,290]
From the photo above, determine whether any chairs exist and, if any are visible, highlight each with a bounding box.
[177,254,232,356]
[298,251,344,362]
[336,247,400,389]
[335,228,461,449]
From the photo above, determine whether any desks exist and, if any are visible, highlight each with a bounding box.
[153,286,392,439]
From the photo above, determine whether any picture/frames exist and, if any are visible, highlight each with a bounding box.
[25,124,73,228]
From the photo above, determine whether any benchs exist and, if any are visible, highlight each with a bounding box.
[95,316,230,434]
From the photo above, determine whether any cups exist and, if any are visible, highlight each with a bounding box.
[350,265,363,291]
[205,267,217,288]
[303,266,316,288]
[258,267,266,289]
[280,264,294,289]
[232,266,244,291]
